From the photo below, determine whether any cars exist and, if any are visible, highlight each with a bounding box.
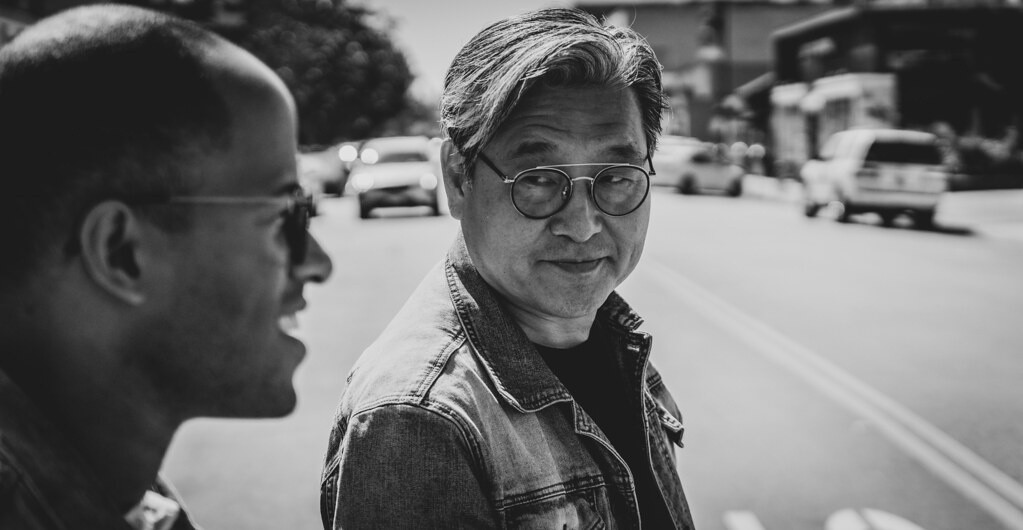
[350,135,441,219]
[648,137,747,198]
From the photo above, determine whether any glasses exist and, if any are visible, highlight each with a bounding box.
[69,192,320,266]
[478,149,656,218]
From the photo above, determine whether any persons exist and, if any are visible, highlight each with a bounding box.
[321,8,696,530]
[0,3,333,530]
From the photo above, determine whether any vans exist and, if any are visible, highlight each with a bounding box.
[799,129,945,227]
